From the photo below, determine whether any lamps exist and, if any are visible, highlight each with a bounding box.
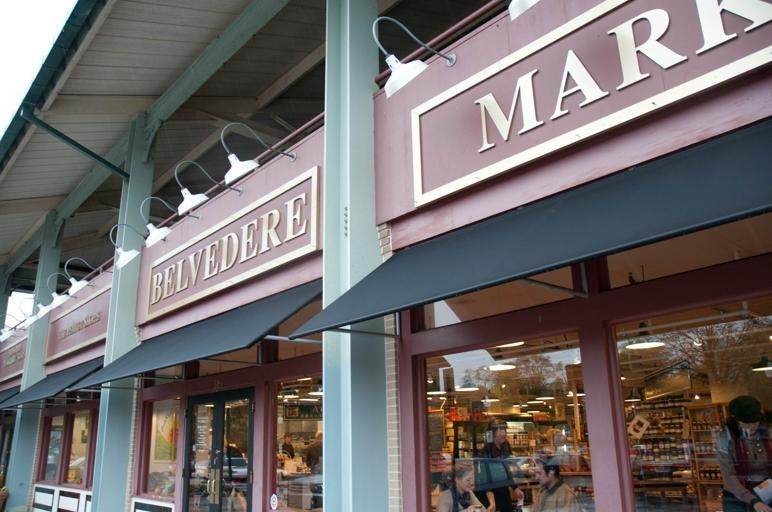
[371,15,459,100]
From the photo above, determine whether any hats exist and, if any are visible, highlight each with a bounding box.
[488,418,507,429]
[728,396,763,423]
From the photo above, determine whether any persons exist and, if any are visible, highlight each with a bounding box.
[480,417,526,512]
[306,430,323,471]
[525,453,584,511]
[433,462,489,512]
[718,395,772,512]
[281,430,295,464]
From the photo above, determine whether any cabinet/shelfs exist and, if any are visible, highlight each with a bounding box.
[624,401,725,511]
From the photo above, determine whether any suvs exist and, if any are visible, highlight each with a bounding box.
[287,466,326,509]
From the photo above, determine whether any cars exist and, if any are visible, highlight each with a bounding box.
[430,451,588,512]
[214,456,251,481]
[632,442,730,462]
